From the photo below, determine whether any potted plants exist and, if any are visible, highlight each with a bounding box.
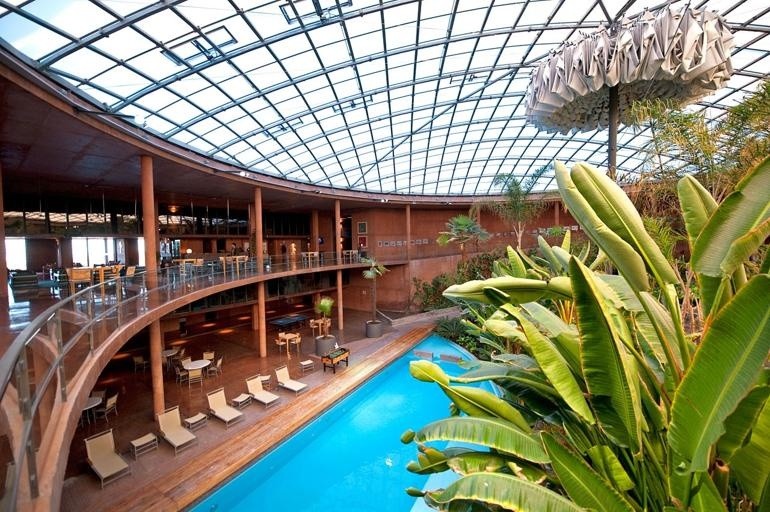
[358,255,389,338]
[308,294,340,358]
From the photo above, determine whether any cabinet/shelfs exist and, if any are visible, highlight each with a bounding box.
[320,347,351,374]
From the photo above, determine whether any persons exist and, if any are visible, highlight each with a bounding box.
[230,242,237,256]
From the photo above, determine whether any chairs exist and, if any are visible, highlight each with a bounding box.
[272,364,310,396]
[245,372,282,409]
[267,311,334,355]
[79,426,132,489]
[204,386,245,431]
[129,345,226,390]
[153,404,201,457]
[172,245,359,279]
[4,259,136,304]
[77,389,120,429]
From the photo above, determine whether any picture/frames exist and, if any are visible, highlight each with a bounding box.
[356,221,367,235]
[357,236,368,249]
[376,238,432,249]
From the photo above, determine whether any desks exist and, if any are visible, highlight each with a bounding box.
[128,431,160,461]
[182,411,208,434]
[299,359,314,376]
[259,374,273,391]
[230,392,253,411]
